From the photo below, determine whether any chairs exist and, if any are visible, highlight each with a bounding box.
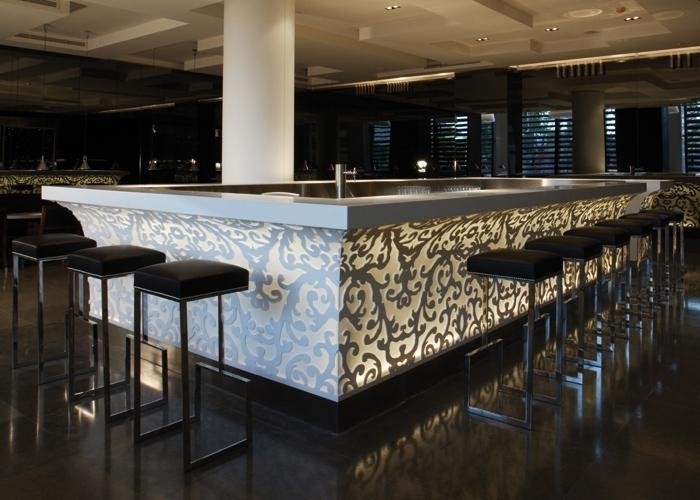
[0,206,47,278]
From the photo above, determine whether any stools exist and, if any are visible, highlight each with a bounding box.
[133,259,253,473]
[64,244,166,425]
[10,232,97,386]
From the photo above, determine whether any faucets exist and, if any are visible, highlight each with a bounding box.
[334,163,358,198]
